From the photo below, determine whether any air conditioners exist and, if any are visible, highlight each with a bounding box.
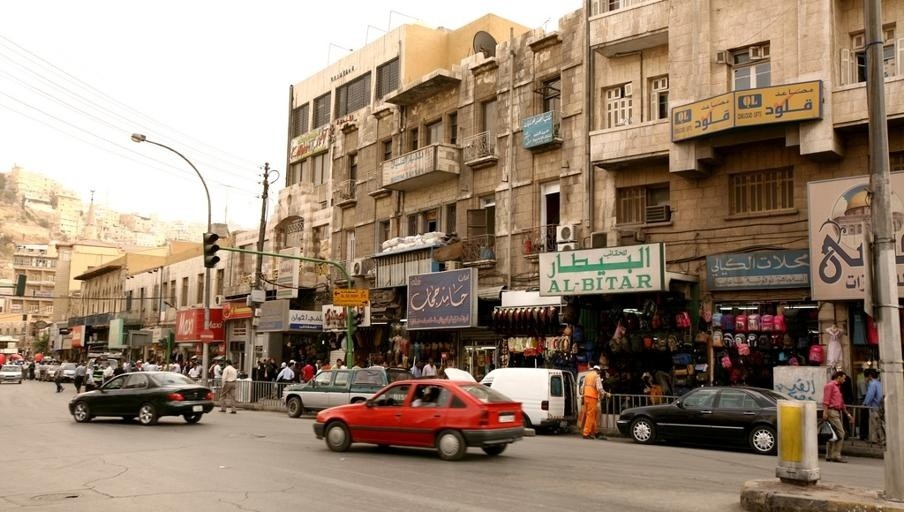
[350,261,368,278]
[557,242,579,251]
[215,295,223,305]
[555,224,578,242]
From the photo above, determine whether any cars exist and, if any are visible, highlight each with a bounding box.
[70,371,215,427]
[0,332,128,391]
[312,380,524,463]
[616,387,797,456]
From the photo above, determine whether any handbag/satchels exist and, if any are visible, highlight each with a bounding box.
[609,311,799,385]
[808,345,825,364]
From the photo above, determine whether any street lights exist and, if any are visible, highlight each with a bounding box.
[131,130,211,388]
[253,159,280,293]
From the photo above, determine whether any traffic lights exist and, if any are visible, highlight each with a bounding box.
[15,274,26,297]
[202,232,219,268]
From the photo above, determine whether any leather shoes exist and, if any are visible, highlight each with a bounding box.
[826,457,847,463]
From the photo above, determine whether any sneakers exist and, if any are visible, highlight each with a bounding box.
[583,432,607,439]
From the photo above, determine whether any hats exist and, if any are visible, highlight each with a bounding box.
[594,365,600,370]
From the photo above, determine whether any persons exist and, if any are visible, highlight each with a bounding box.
[824,324,845,370]
[208,358,240,387]
[351,357,438,392]
[6,358,65,393]
[252,358,347,393]
[70,359,114,393]
[113,359,202,382]
[218,359,237,414]
[577,359,611,440]
[822,368,886,463]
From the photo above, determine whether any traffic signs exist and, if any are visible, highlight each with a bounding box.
[333,289,368,307]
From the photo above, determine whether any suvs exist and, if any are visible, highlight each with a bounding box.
[280,368,417,420]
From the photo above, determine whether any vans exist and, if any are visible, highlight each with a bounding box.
[444,367,578,430]
